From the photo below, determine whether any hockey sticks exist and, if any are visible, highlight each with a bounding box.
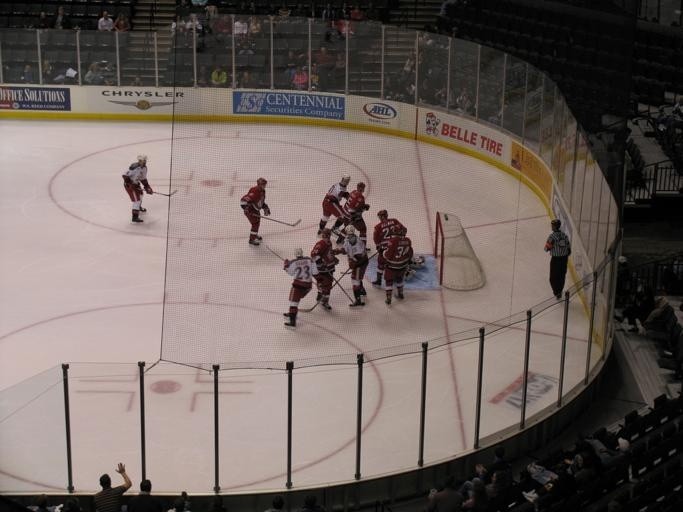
[249,214,302,226]
[340,251,378,275]
[139,189,177,196]
[297,268,350,312]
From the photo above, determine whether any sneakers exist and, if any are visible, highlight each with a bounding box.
[285,321,295,326]
[249,240,259,245]
[320,301,331,310]
[331,225,341,235]
[132,217,143,222]
[372,279,404,304]
[349,301,362,306]
[140,207,146,211]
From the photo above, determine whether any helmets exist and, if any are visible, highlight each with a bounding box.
[377,210,388,217]
[322,228,331,238]
[137,153,147,160]
[552,218,561,227]
[357,182,365,188]
[345,225,355,235]
[342,174,350,180]
[257,178,267,185]
[294,247,303,257]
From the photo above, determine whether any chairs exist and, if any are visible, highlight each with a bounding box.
[1,1,681,110]
[492,110,682,512]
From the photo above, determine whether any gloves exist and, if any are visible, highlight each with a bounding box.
[262,204,271,216]
[144,184,152,194]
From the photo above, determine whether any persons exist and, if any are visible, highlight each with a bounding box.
[544,218,570,299]
[392,3,507,118]
[238,178,270,245]
[14,1,363,90]
[9,462,326,511]
[613,286,655,333]
[120,154,152,223]
[284,176,425,326]
[429,436,631,511]
